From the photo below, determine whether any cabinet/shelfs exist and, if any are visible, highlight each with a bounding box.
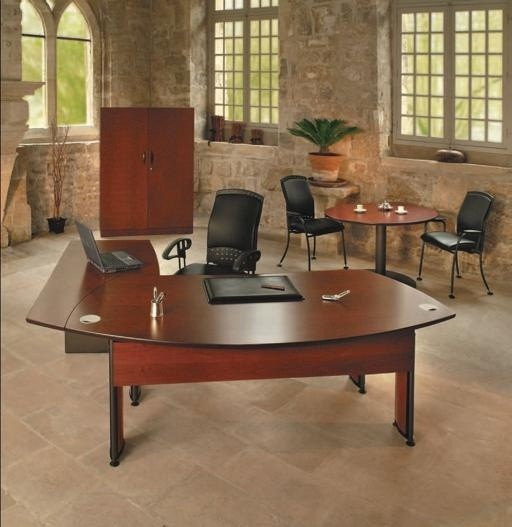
[98,106,194,236]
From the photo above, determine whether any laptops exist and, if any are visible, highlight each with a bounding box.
[75,220,145,273]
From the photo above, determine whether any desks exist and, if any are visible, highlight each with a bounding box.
[23,238,456,468]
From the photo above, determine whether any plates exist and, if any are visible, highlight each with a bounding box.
[353,209,367,213]
[395,210,407,215]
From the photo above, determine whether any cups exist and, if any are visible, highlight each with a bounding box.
[150,300,164,319]
[398,206,404,212]
[357,205,363,210]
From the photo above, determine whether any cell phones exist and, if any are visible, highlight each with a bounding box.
[322,290,350,301]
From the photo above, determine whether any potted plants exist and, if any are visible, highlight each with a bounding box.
[288,116,363,188]
[45,117,69,234]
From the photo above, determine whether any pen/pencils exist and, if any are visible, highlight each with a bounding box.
[153,286,166,302]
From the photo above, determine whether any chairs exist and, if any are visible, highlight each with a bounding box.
[161,189,264,276]
[278,176,495,299]
